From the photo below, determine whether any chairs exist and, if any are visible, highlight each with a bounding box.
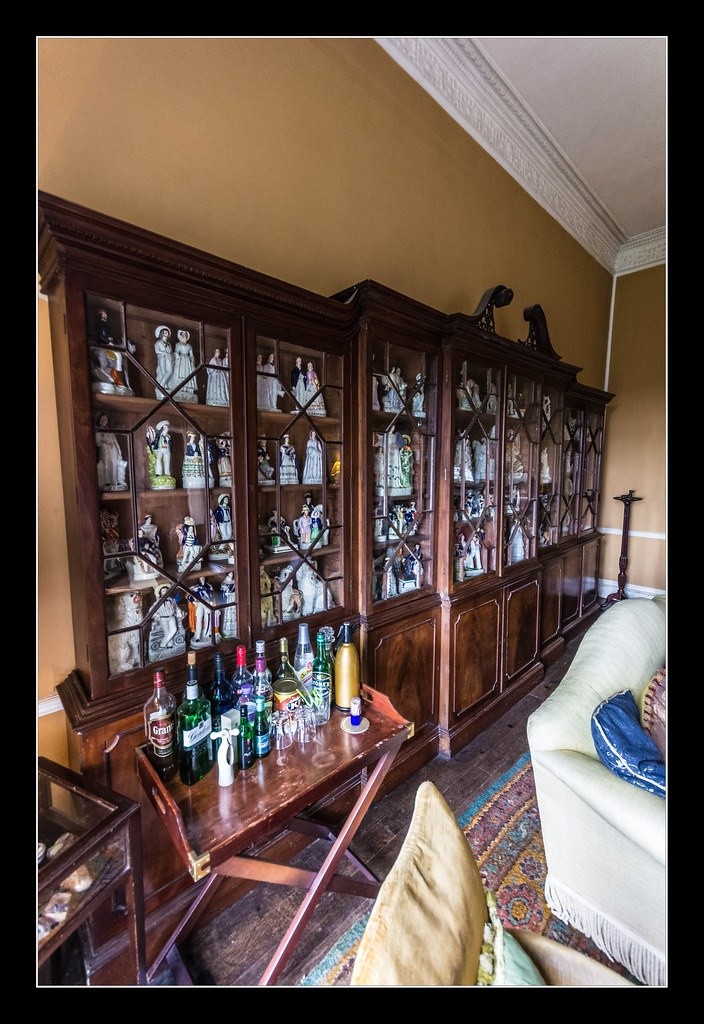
[350,779,639,987]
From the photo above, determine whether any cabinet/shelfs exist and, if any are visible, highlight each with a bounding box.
[37,190,617,986]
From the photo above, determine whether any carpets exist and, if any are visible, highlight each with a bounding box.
[299,750,651,987]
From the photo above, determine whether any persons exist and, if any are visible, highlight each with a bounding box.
[90,308,113,369]
[94,412,124,482]
[378,543,423,596]
[155,330,198,391]
[147,420,172,475]
[258,430,322,479]
[291,355,324,408]
[265,492,330,542]
[371,349,425,410]
[154,578,214,642]
[458,517,556,582]
[178,492,232,561]
[220,558,335,627]
[461,372,530,411]
[375,501,418,535]
[186,433,233,473]
[455,427,551,483]
[100,512,161,566]
[257,353,285,408]
[373,435,413,488]
[208,348,229,399]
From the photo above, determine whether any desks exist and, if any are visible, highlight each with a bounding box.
[37,755,147,986]
[135,683,416,985]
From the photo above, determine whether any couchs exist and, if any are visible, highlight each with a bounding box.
[526,593,666,987]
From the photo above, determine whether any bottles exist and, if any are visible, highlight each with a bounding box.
[143,622,361,785]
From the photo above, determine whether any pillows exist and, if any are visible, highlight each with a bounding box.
[350,779,490,986]
[639,662,666,765]
[591,689,666,799]
[476,892,549,986]
